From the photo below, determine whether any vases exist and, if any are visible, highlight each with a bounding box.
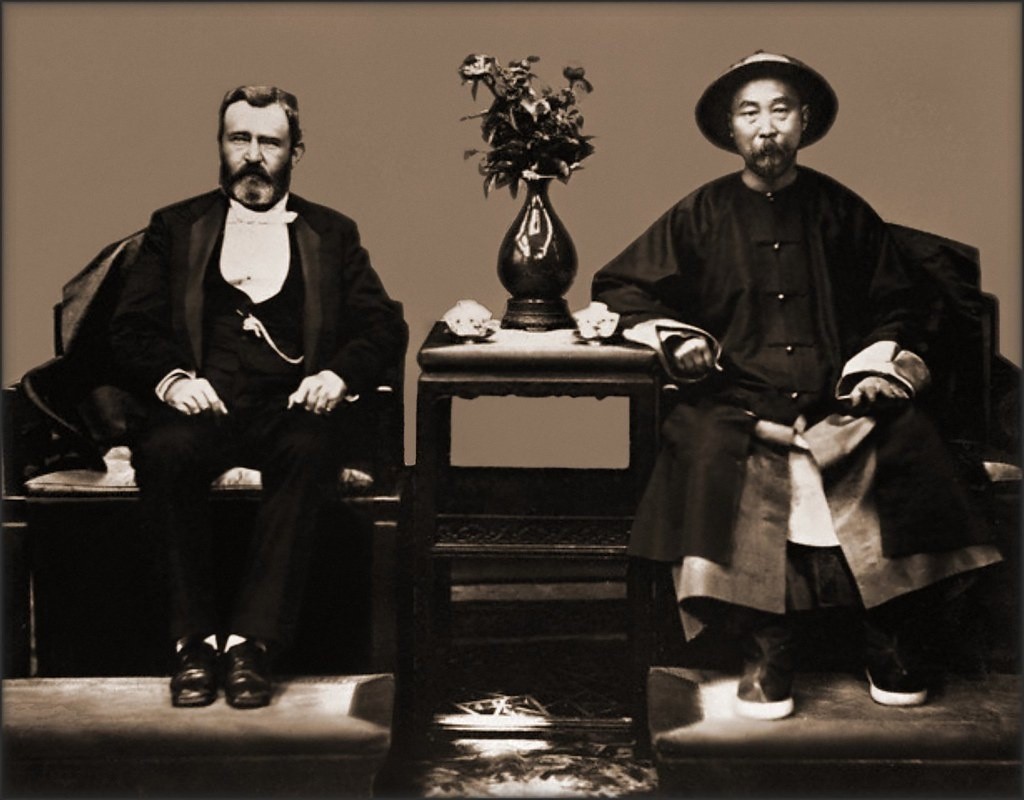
[495,173,581,329]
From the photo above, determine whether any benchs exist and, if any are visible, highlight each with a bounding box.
[648,664,1024,800]
[0,670,398,799]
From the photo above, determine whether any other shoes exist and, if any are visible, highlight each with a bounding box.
[737,624,797,719]
[865,626,928,707]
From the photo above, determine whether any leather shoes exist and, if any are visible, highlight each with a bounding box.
[170,640,221,706]
[222,637,272,706]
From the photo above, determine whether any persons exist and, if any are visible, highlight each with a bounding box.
[124,82,410,706]
[591,50,1005,719]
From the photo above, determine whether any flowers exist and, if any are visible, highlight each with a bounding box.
[458,53,596,199]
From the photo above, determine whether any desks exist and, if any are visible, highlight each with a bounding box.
[407,317,664,757]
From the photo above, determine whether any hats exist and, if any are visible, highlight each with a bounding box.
[695,51,839,153]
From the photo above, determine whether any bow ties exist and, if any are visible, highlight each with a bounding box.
[228,198,299,224]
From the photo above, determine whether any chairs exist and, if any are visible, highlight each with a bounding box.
[893,223,1024,657]
[1,228,404,686]
